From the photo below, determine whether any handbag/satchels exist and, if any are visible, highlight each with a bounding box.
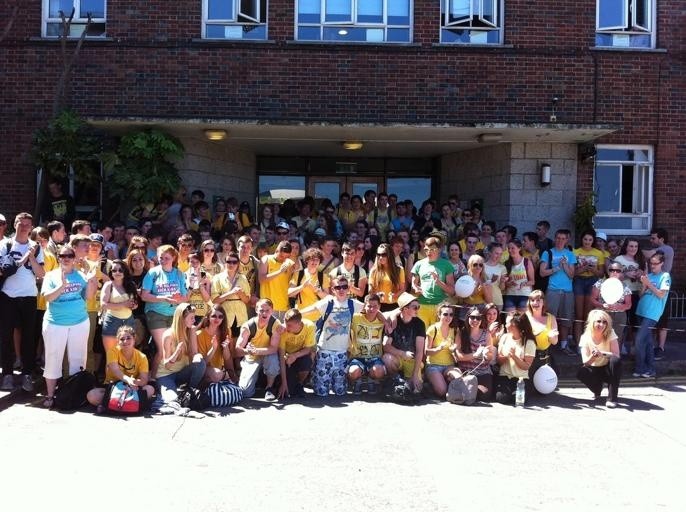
[175,382,210,409]
[205,380,244,407]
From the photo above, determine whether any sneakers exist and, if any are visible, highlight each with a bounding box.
[265,391,275,400]
[43,398,54,408]
[629,347,636,359]
[653,346,666,361]
[0,374,14,390]
[96,404,104,413]
[640,369,656,378]
[568,339,576,349]
[21,374,35,391]
[353,380,362,395]
[621,343,628,356]
[368,380,377,394]
[294,383,306,397]
[12,359,21,368]
[633,371,644,377]
[606,400,618,407]
[559,343,577,357]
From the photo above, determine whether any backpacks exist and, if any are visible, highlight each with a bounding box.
[534,249,553,294]
[102,380,140,416]
[315,299,353,341]
[52,366,98,411]
[446,370,478,405]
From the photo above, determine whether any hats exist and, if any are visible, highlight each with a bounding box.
[275,221,290,231]
[596,232,608,242]
[397,293,418,309]
[88,233,104,247]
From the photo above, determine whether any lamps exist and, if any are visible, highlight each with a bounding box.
[341,141,364,150]
[540,162,552,186]
[203,128,229,142]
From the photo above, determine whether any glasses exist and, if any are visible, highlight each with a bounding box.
[413,305,481,320]
[58,254,74,258]
[110,268,123,272]
[609,269,622,273]
[211,315,223,318]
[462,213,470,217]
[334,285,348,290]
[376,253,386,257]
[355,247,364,250]
[276,231,289,235]
[180,243,238,264]
[448,202,455,205]
[474,263,483,267]
[183,303,193,314]
[424,247,439,252]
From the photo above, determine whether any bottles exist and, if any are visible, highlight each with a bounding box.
[516,376,525,407]
